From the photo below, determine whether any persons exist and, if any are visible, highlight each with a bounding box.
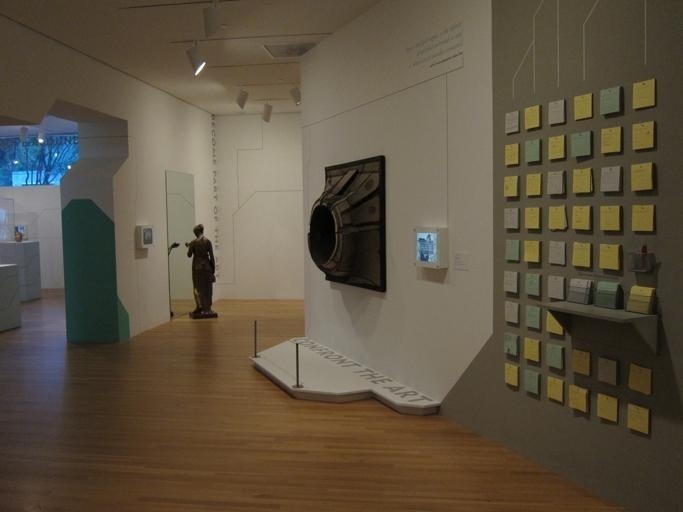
[185,224,218,319]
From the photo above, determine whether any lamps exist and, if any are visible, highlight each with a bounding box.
[21,126,47,144]
[289,86,302,106]
[262,100,273,123]
[235,88,248,109]
[184,42,206,77]
